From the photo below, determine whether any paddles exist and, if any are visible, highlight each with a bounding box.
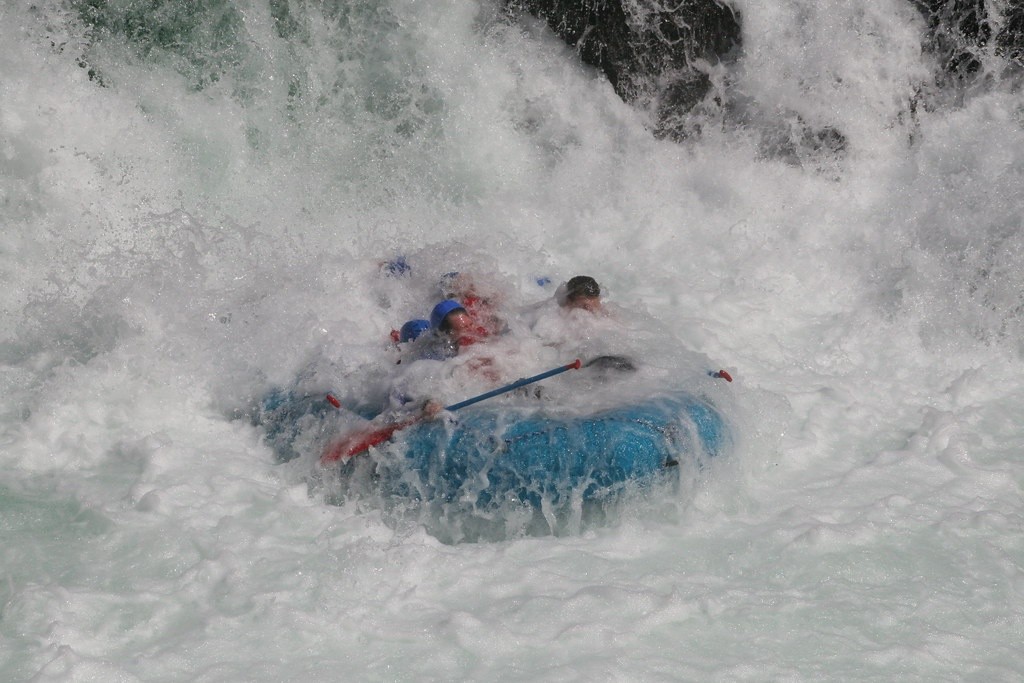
[323,358,582,463]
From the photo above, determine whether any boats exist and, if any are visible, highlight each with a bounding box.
[262,257,721,510]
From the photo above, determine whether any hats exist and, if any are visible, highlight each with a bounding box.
[565,276,601,301]
[400,319,430,343]
[429,300,465,329]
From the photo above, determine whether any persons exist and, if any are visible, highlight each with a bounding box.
[383,254,601,418]
